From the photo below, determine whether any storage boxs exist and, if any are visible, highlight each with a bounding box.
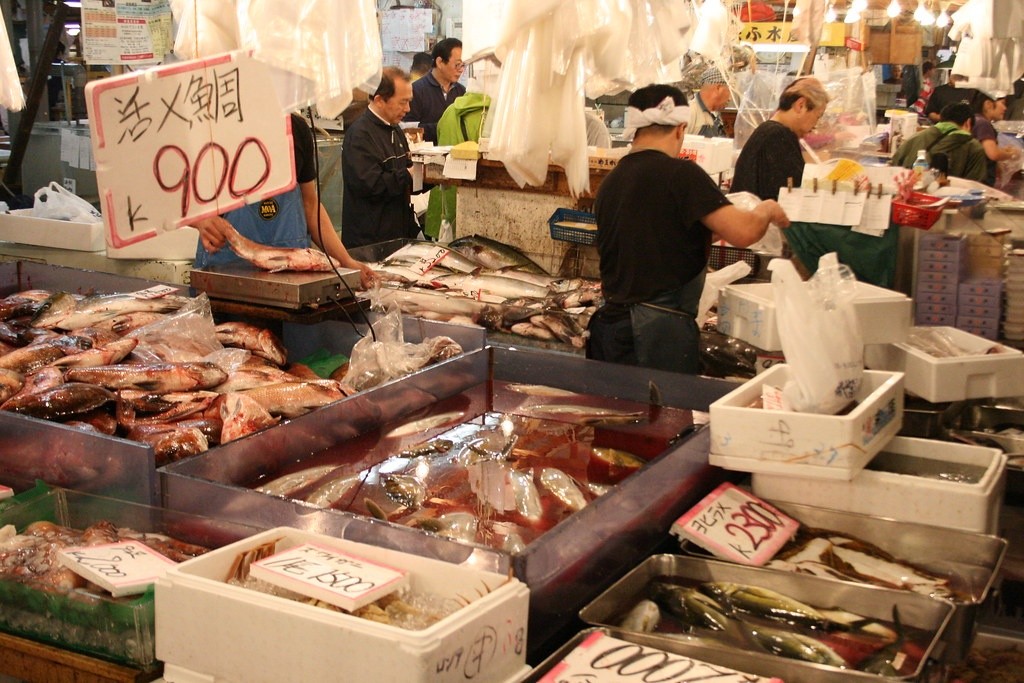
[0,133,1024,683]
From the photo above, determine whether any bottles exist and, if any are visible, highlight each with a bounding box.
[912,149,930,172]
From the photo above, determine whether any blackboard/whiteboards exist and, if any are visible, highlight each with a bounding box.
[83,44,297,249]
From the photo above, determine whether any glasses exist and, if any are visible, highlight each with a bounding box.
[455,64,465,70]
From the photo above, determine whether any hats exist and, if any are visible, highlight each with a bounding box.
[700,67,736,87]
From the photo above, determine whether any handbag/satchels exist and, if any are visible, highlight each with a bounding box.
[768,253,864,416]
[32,181,103,225]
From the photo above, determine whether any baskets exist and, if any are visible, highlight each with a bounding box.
[547,208,598,245]
[892,193,943,230]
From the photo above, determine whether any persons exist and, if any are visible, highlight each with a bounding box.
[883,62,1024,188]
[402,37,492,146]
[342,67,423,262]
[729,78,830,202]
[586,112,611,148]
[188,114,381,292]
[684,66,732,137]
[587,83,790,375]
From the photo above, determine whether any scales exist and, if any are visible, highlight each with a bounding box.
[188,260,363,313]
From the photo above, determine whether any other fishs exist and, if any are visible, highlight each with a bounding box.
[0,284,359,466]
[219,218,606,353]
[255,380,952,674]
